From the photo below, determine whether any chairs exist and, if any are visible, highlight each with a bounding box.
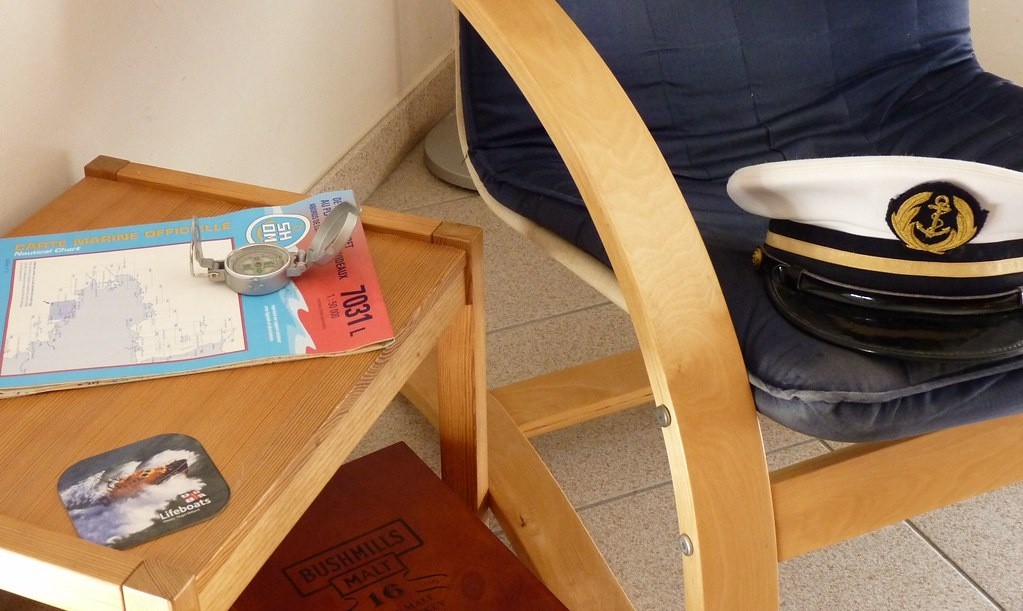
[401,0,1023,611]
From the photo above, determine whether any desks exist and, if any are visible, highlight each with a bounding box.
[0,154,488,611]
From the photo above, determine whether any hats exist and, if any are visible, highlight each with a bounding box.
[725,155,1023,358]
